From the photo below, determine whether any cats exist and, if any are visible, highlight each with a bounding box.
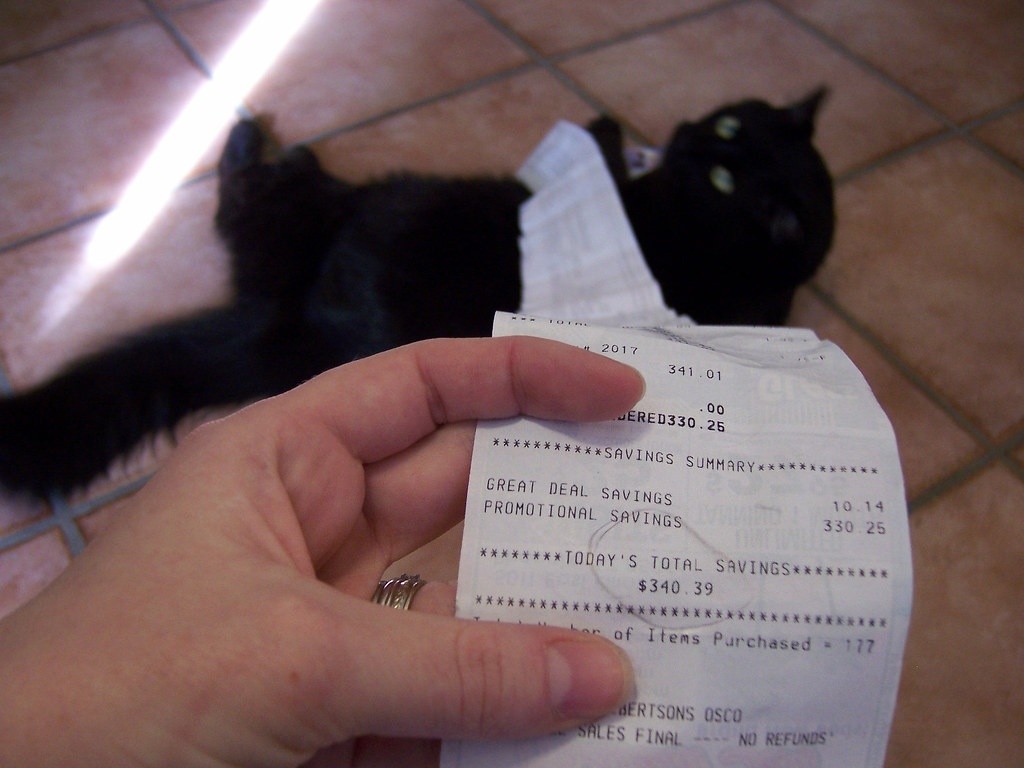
[0,87,844,513]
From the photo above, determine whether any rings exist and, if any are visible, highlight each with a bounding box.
[370,574,428,611]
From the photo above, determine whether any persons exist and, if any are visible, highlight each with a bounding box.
[0,335,646,768]
